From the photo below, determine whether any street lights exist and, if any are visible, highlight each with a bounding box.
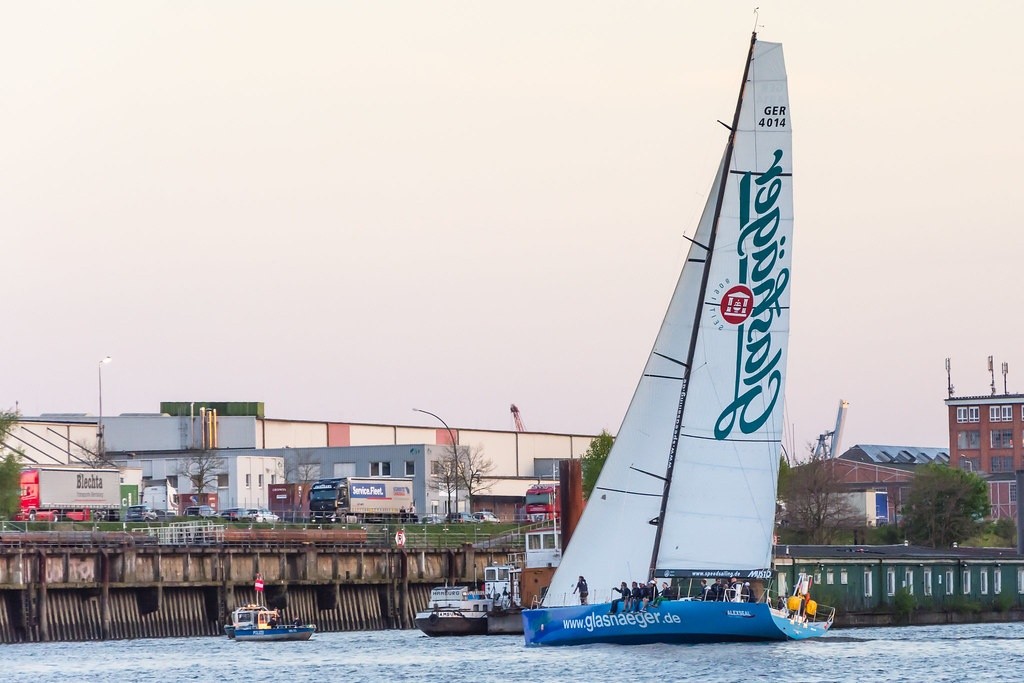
[99,356,110,452]
[413,408,459,523]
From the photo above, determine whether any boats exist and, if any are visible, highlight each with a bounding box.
[224,612,235,638]
[234,571,316,642]
[415,579,493,638]
[484,565,524,633]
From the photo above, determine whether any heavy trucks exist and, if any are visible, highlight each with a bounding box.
[522,484,560,522]
[306,477,413,524]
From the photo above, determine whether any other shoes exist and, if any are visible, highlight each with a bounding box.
[607,607,647,614]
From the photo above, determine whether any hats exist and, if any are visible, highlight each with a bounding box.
[648,580,655,585]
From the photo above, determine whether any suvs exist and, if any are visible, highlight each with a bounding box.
[183,504,221,519]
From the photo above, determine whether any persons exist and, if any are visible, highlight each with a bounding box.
[622,582,640,613]
[606,582,632,615]
[768,592,805,620]
[292,617,301,629]
[701,579,709,602]
[724,578,756,601]
[572,576,588,606]
[400,505,407,523]
[267,616,277,629]
[407,502,416,523]
[711,578,724,601]
[631,580,648,613]
[237,614,247,622]
[640,581,658,613]
[648,581,671,608]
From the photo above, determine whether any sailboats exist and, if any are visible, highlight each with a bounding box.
[522,2,836,644]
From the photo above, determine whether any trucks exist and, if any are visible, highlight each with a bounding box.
[141,484,179,516]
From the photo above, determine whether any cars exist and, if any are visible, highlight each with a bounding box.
[16,508,59,522]
[470,512,500,523]
[126,505,158,522]
[220,508,246,520]
[418,517,435,525]
[94,509,106,518]
[242,508,281,523]
[104,508,118,520]
